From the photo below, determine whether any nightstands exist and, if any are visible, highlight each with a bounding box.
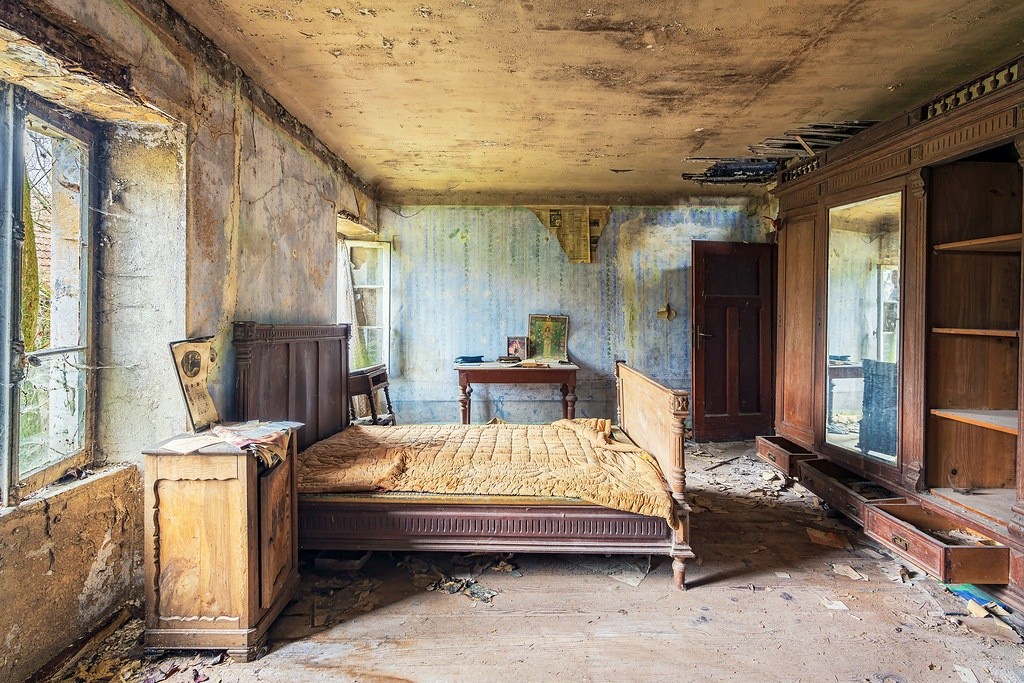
[349,364,397,426]
[140,421,305,664]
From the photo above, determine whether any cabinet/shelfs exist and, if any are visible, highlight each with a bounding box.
[754,57,1024,614]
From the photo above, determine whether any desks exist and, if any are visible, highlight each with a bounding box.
[828,360,863,425]
[453,360,581,424]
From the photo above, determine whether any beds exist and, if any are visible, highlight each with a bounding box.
[231,320,695,591]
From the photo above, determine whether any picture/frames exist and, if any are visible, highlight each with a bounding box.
[506,336,527,361]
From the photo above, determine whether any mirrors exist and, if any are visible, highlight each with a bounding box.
[825,191,901,466]
